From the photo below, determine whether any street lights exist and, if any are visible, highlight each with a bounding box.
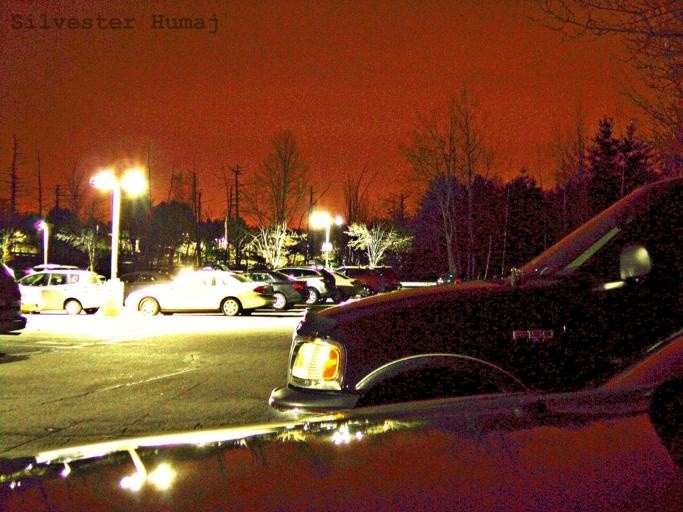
[35,219,50,271]
[306,210,346,273]
[95,165,151,278]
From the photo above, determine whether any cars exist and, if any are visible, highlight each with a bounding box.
[435,273,471,288]
[0,324,683,508]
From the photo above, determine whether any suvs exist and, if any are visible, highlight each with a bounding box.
[269,178,683,418]
